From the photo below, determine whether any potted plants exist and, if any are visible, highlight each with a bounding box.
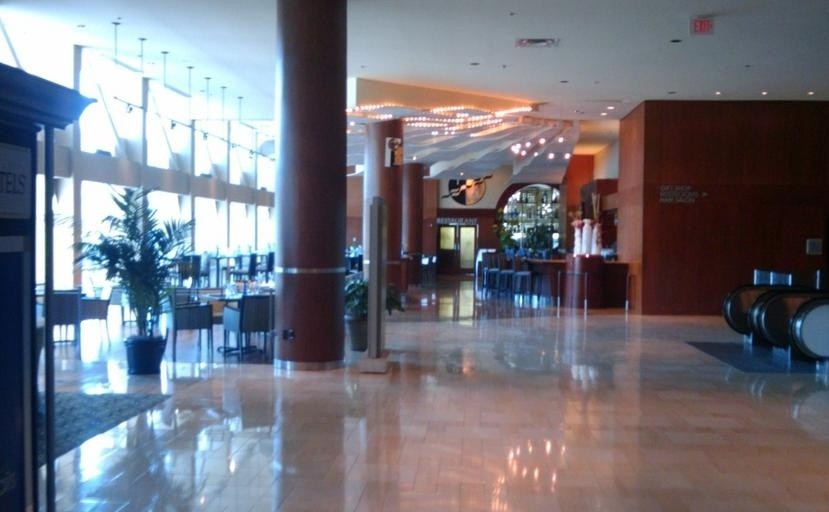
[69,183,195,375]
[343,278,372,351]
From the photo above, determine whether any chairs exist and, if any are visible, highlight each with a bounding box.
[476,250,544,309]
[53,249,276,363]
